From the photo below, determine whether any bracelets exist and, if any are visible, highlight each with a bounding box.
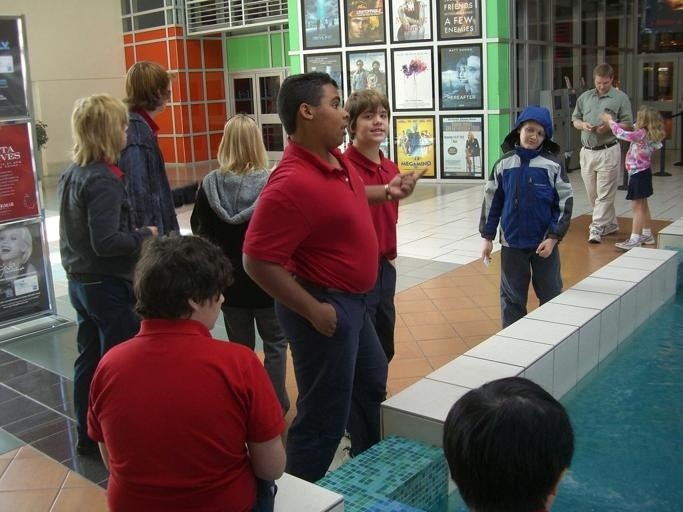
[384,183,393,201]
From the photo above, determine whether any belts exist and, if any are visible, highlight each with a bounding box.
[585,142,616,150]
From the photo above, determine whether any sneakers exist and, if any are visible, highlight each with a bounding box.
[614,238,641,250]
[601,222,618,236]
[640,233,655,245]
[588,224,602,243]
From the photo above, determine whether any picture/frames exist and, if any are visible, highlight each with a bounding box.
[300,0,484,179]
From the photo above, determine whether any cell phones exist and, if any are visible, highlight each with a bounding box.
[592,126,598,129]
[484,251,489,266]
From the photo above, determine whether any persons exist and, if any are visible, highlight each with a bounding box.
[368,61,385,92]
[442,376,574,512]
[86,234,287,512]
[464,54,481,100]
[349,17,373,40]
[0,227,38,290]
[397,0,425,41]
[189,113,291,416]
[571,63,634,243]
[116,59,202,241]
[400,123,430,155]
[599,104,666,251]
[340,89,400,440]
[465,132,480,172]
[456,56,471,93]
[56,93,159,456]
[479,104,574,331]
[240,71,429,484]
[351,59,367,93]
